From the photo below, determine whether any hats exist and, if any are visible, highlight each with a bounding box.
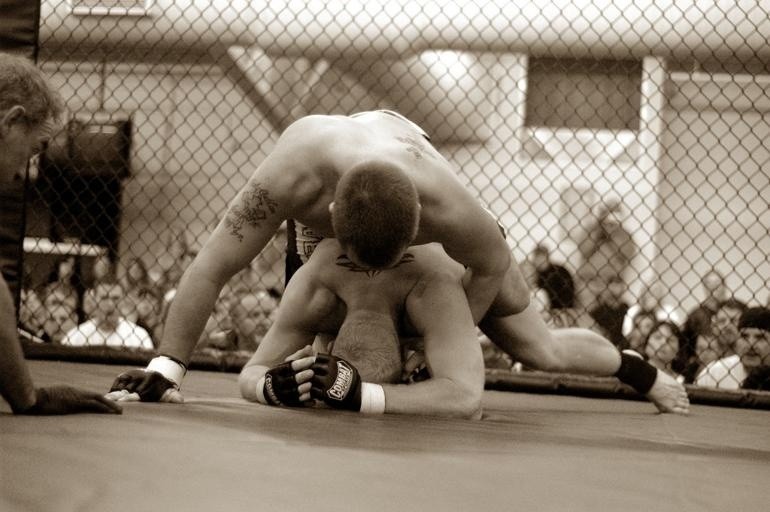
[736,305,770,333]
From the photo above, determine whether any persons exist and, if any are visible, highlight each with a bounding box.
[237,204,692,415]
[0,50,125,416]
[98,108,532,406]
[0,198,769,396]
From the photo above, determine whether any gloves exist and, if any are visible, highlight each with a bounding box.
[308,349,389,419]
[255,358,305,411]
[12,382,126,417]
[109,366,185,404]
